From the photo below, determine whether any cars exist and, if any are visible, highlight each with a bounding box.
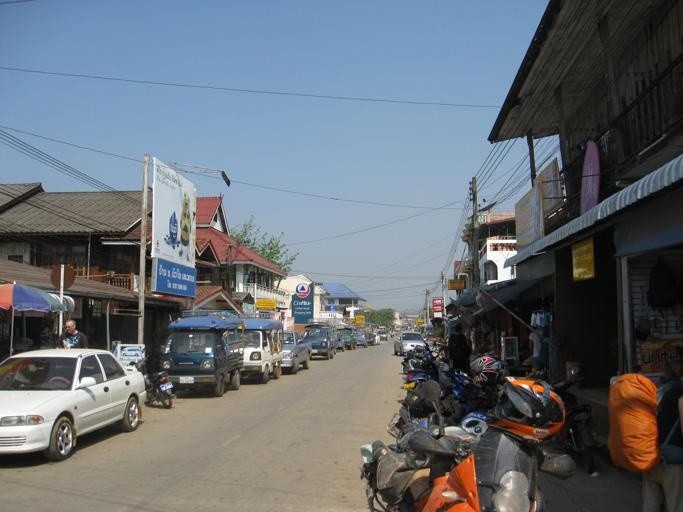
[0,347,146,460]
[157,314,388,398]
[393,331,426,357]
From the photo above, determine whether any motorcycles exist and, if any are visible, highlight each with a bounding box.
[359,344,592,512]
[130,346,174,409]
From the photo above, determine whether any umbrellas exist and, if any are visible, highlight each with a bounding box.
[0,280,65,357]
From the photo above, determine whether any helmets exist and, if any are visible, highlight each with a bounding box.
[485,376,565,444]
[469,355,509,389]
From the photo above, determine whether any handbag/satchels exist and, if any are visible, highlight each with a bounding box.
[400,382,444,417]
[364,443,434,508]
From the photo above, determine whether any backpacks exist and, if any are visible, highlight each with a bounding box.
[606,372,664,474]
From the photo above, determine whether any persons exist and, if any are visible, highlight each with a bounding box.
[447,323,472,371]
[639,356,683,511]
[54,319,88,349]
[526,324,544,376]
[175,336,191,354]
[196,335,213,355]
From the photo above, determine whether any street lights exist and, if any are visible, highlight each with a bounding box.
[137,150,233,359]
